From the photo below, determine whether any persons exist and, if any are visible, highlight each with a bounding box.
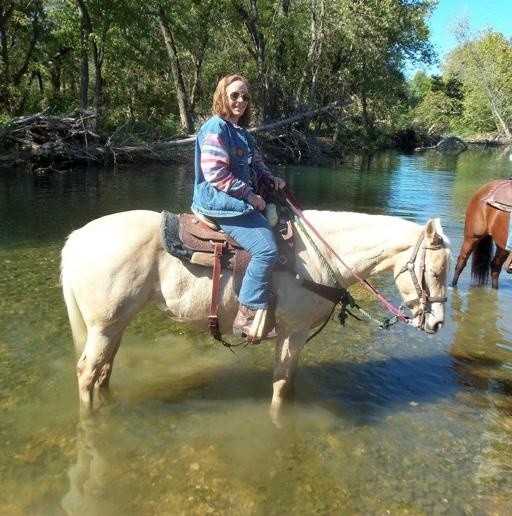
[190,72,287,339]
[503,212,512,273]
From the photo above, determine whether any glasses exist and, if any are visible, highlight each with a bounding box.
[230,92,250,101]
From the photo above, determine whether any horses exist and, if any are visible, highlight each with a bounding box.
[450,179,512,290]
[60,210,451,427]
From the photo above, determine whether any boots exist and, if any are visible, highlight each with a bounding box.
[233,303,279,338]
